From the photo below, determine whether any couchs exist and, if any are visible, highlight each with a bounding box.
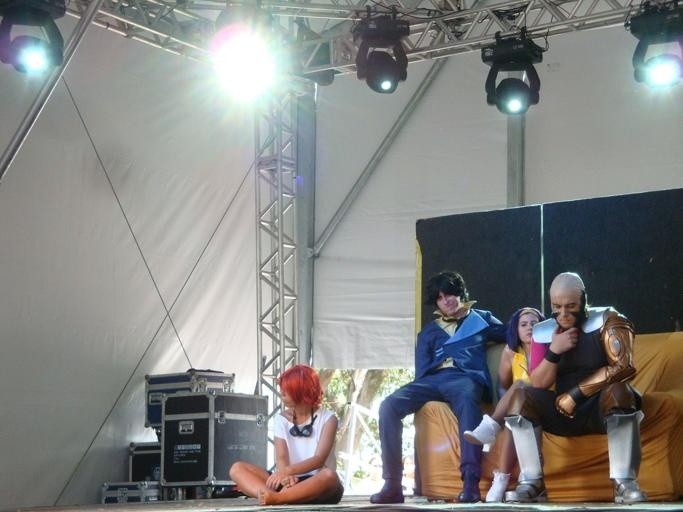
[416,331,683,501]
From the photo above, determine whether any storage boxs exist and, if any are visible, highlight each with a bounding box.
[129,441,161,482]
[101,481,162,505]
[143,371,235,427]
[160,393,269,487]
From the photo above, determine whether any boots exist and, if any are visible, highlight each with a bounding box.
[485,468,510,503]
[460,414,502,452]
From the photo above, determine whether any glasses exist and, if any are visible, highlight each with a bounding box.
[288,415,318,437]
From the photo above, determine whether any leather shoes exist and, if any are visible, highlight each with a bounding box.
[370,483,405,503]
[457,481,481,504]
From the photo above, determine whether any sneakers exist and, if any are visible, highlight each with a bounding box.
[611,478,646,503]
[505,476,551,503]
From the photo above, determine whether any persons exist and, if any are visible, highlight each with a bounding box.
[229,365,344,506]
[370,271,507,503]
[464,307,557,503]
[504,272,648,505]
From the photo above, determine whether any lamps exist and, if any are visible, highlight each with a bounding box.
[625,4,683,91]
[352,4,411,95]
[482,29,546,117]
[1,2,68,78]
